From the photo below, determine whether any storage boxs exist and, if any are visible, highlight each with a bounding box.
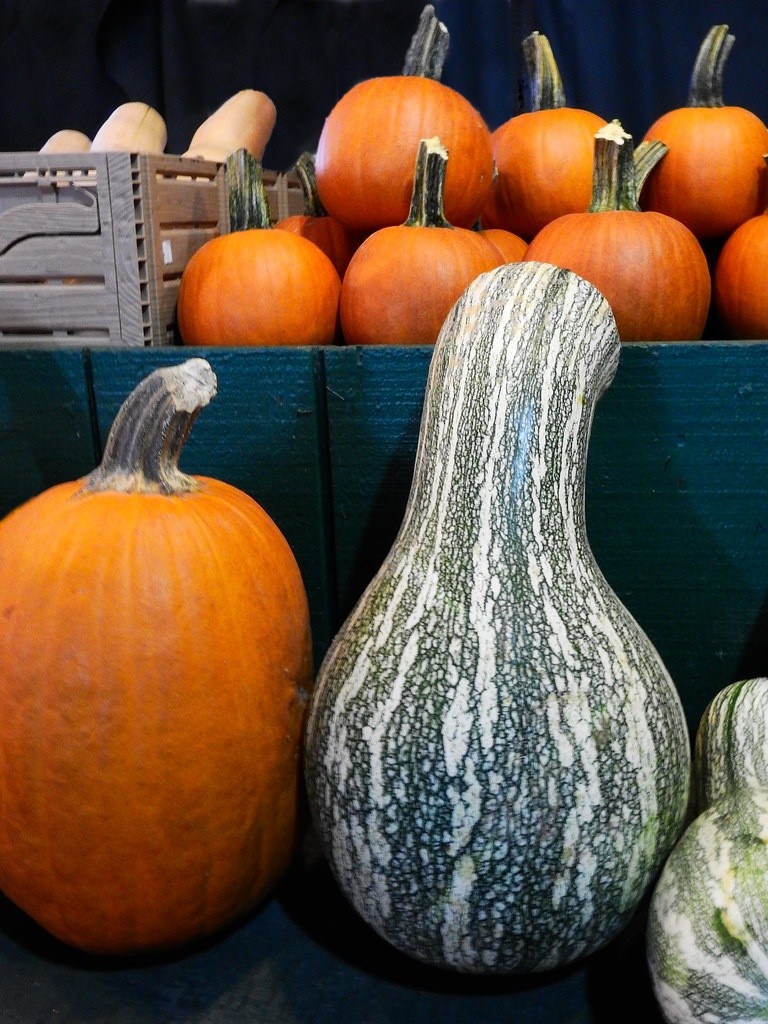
[0,153,768,1024]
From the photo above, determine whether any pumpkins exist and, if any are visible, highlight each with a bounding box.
[0,5,768,1024]
